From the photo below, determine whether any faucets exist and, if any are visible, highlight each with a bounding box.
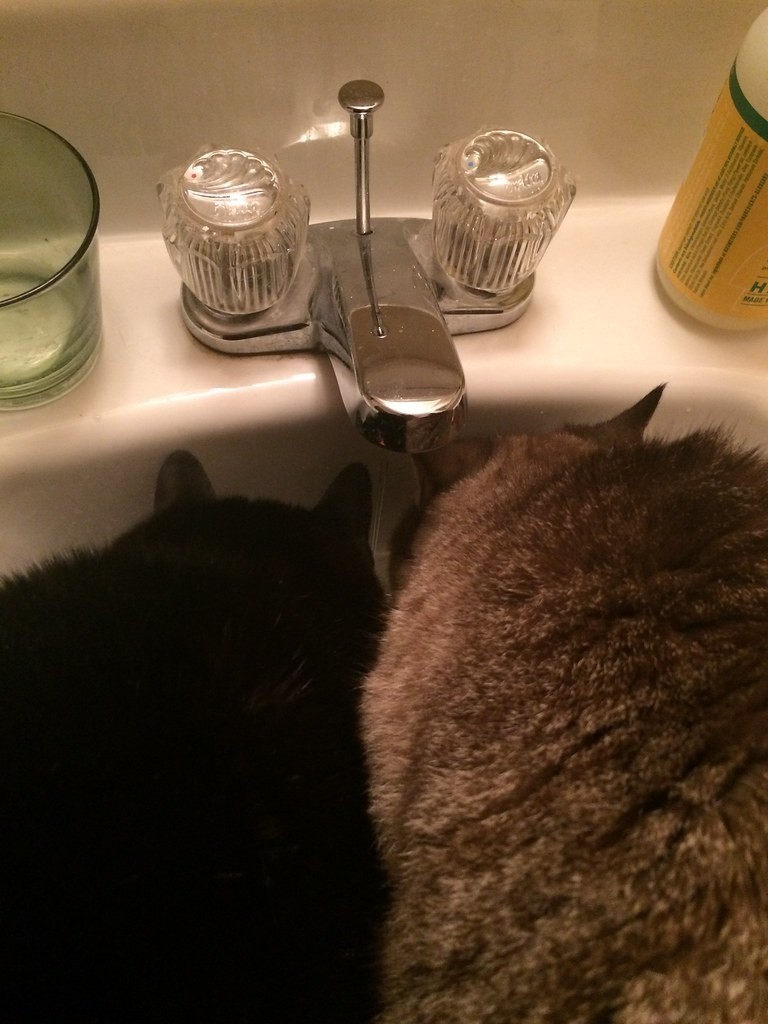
[154,75,579,458]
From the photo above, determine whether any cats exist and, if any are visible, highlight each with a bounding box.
[0,383,768,1024]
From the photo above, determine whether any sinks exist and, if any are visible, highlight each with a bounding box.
[2,193,768,578]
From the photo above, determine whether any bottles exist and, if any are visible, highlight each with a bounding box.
[654,6,768,335]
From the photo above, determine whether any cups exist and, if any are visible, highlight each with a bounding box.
[1,109,101,415]
